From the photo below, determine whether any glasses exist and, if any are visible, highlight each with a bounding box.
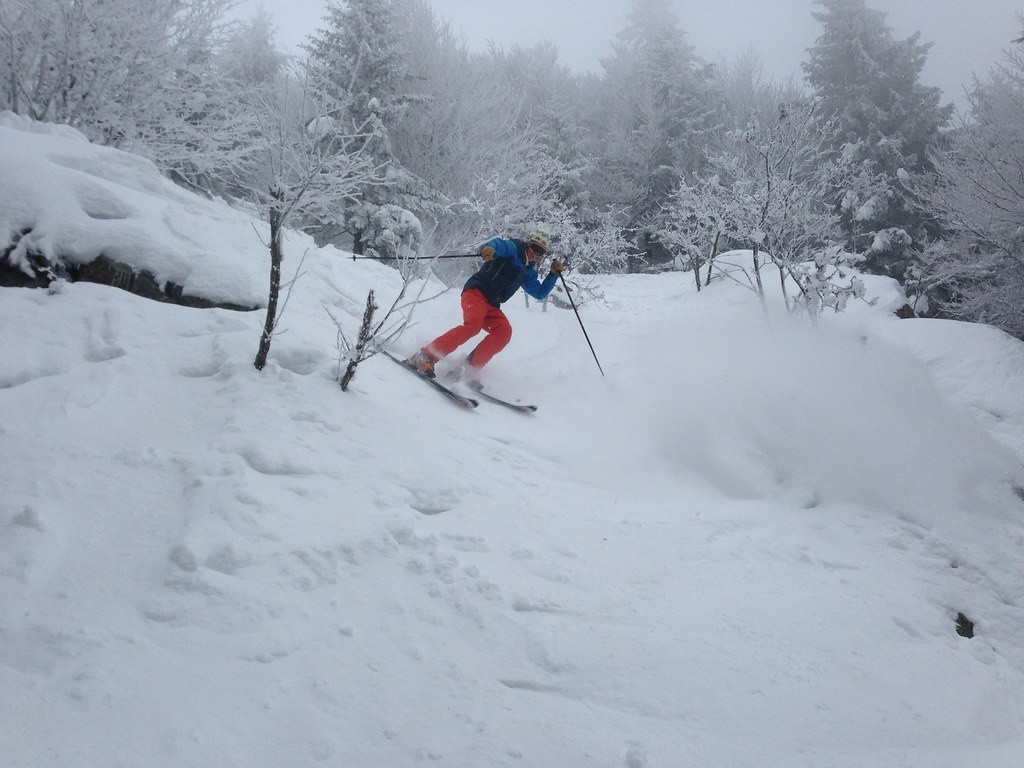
[530,243,546,256]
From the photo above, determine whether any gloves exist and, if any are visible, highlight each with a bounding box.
[551,260,563,275]
[482,246,496,262]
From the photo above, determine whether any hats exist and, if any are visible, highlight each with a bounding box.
[529,230,550,249]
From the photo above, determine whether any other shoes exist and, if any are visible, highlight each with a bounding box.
[411,352,435,378]
[458,365,483,390]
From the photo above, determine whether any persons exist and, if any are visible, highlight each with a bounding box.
[406,228,563,390]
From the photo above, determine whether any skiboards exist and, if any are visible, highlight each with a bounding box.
[381,350,538,413]
